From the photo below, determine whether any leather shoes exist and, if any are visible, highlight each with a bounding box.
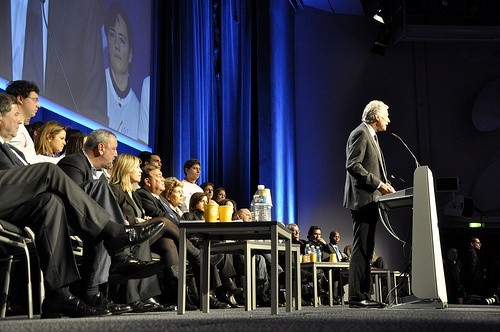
[220,277,243,293]
[256,278,267,286]
[209,291,231,308]
[225,295,246,309]
[81,292,132,315]
[348,299,386,309]
[40,296,112,319]
[107,256,163,282]
[103,220,165,261]
[130,297,173,311]
[301,299,313,306]
[186,301,199,311]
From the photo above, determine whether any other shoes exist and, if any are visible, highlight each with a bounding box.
[194,254,224,273]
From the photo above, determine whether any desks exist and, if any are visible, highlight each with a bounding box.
[177,221,411,315]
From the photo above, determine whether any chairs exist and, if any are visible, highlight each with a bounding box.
[0,219,161,319]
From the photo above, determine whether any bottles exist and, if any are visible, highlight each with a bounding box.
[315,246,321,262]
[251,185,273,221]
[305,244,311,262]
[311,245,316,253]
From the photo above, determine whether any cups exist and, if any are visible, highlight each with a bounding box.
[330,254,337,262]
[219,206,233,222]
[302,253,317,263]
[204,204,218,222]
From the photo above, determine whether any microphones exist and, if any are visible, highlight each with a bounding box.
[392,133,420,168]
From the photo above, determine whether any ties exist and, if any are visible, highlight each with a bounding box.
[335,247,342,261]
[158,198,178,220]
[374,135,379,144]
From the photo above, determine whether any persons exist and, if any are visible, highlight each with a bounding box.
[212,187,226,203]
[5,80,41,165]
[178,159,205,215]
[138,152,162,170]
[181,192,245,308]
[55,129,175,315]
[24,115,117,183]
[138,76,151,145]
[444,247,464,304]
[134,164,232,309]
[108,153,224,312]
[287,223,387,306]
[162,176,185,219]
[0,0,111,129]
[342,100,396,309]
[232,208,284,308]
[0,88,166,318]
[200,182,214,200]
[104,4,141,142]
[218,199,267,286]
[203,199,271,307]
[464,236,488,302]
